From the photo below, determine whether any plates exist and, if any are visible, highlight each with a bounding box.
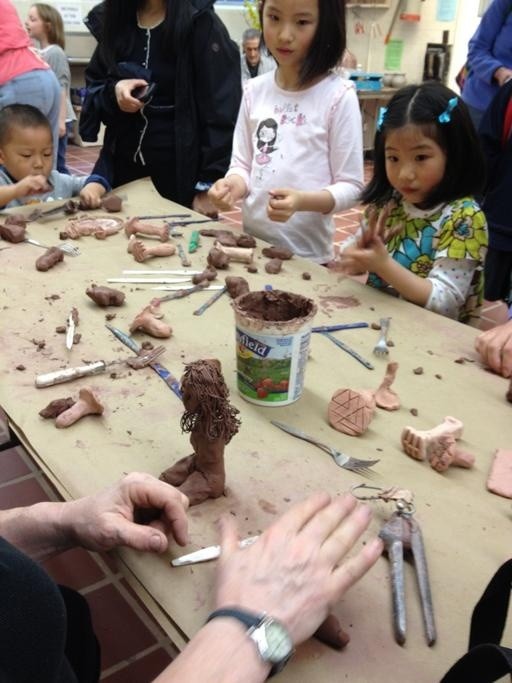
[75,214,121,236]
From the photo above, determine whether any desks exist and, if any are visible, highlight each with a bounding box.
[0,177,511,681]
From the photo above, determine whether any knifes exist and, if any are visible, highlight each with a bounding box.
[310,320,369,333]
[107,322,184,399]
[322,331,377,371]
[107,266,227,291]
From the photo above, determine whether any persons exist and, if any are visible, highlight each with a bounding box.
[160,355,237,509]
[23,0,80,179]
[332,81,487,330]
[1,0,62,173]
[78,0,245,218]
[239,27,278,82]
[453,0,510,135]
[470,75,512,379]
[0,467,393,683]
[0,101,113,216]
[207,0,368,273]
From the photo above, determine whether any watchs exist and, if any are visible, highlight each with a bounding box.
[200,606,298,681]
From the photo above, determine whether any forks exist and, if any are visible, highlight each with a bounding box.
[23,236,81,256]
[373,316,395,357]
[263,417,382,470]
[34,344,165,392]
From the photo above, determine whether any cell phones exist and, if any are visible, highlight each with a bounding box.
[130,83,155,100]
